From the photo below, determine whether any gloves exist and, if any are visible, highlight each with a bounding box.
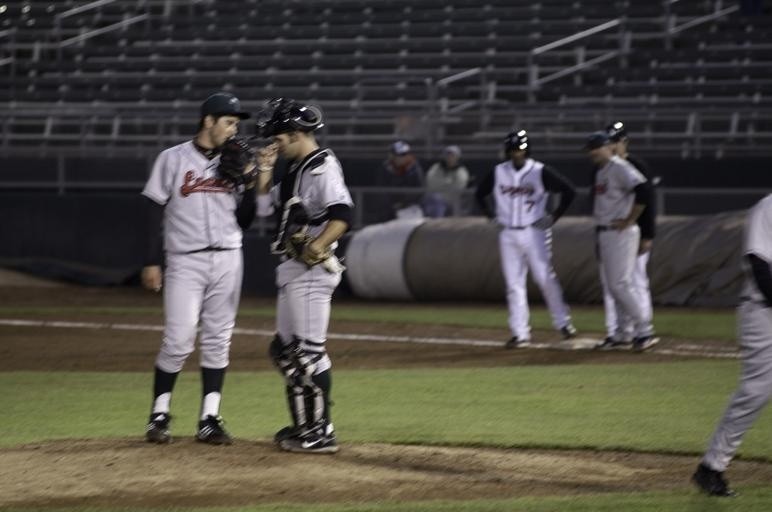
[533,215,554,230]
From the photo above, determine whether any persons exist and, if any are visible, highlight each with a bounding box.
[425,145,472,215]
[137,93,257,446]
[255,96,357,453]
[584,131,660,352]
[477,128,579,350]
[691,192,771,494]
[590,120,656,349]
[375,140,423,218]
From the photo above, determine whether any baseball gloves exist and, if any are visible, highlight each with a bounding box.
[286,233,330,266]
[215,135,259,191]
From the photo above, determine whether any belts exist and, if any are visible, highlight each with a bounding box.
[597,225,613,231]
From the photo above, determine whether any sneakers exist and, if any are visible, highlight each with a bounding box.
[275,424,339,453]
[506,324,659,351]
[198,415,231,444]
[692,466,739,497]
[146,414,171,443]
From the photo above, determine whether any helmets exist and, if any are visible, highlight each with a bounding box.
[257,97,324,137]
[504,131,527,150]
[606,123,626,142]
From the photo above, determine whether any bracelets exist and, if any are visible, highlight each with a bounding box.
[258,163,274,171]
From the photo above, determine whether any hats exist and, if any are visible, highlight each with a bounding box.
[200,93,248,119]
[583,132,609,151]
